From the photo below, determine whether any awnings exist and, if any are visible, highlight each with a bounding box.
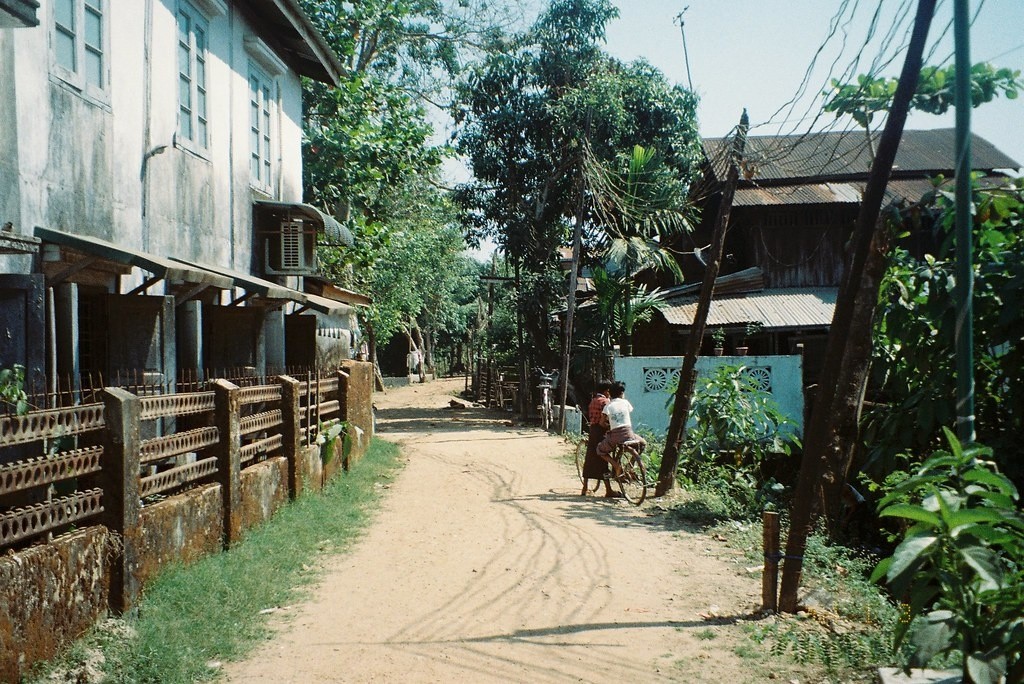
[33,226,234,296]
[167,258,307,306]
[288,282,372,318]
[255,200,354,247]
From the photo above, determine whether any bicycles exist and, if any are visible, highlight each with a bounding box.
[535,365,559,431]
[575,421,662,505]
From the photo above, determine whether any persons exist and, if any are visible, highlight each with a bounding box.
[596,380,647,480]
[581,379,622,497]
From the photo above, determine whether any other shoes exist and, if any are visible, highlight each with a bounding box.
[605,491,626,497]
[582,488,588,496]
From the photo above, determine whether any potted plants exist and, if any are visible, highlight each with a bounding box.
[711,327,726,356]
[735,320,764,355]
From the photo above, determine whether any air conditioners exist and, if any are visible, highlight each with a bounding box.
[281,221,316,273]
